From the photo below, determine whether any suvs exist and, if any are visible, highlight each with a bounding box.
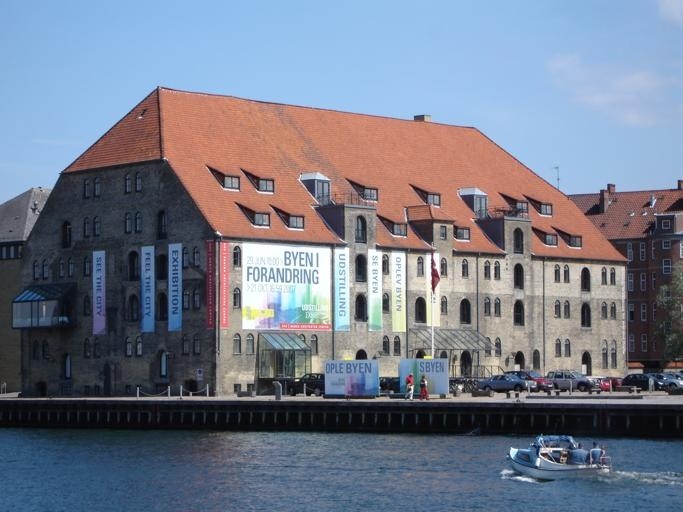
[504,370,554,392]
[546,369,594,392]
[287,372,324,397]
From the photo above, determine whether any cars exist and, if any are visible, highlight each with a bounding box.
[447,375,478,393]
[586,371,682,395]
[477,375,537,392]
[379,376,400,394]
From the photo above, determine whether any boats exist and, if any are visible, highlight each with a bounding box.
[507,434,613,480]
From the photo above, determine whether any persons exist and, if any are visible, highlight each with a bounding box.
[567,440,587,465]
[404,373,414,401]
[419,374,429,400]
[592,440,602,464]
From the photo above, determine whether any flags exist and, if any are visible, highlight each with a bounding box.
[431,250,440,295]
